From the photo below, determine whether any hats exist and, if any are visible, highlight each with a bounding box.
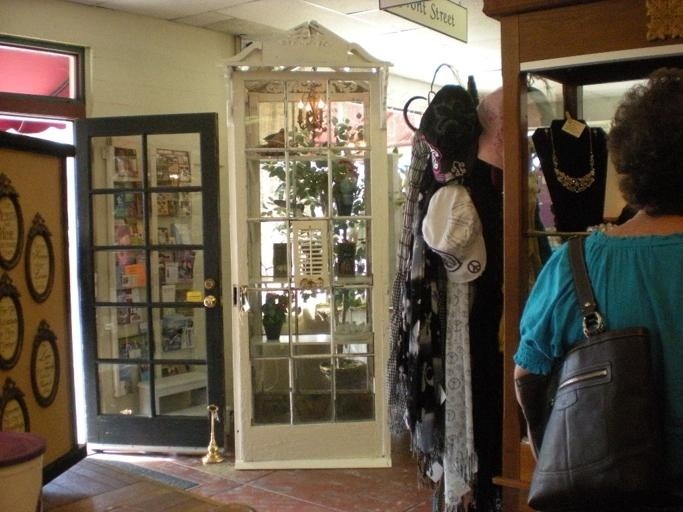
[476,86,509,171]
[419,183,489,286]
[420,136,473,183]
[420,83,478,154]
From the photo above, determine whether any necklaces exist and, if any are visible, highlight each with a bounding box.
[549,125,596,194]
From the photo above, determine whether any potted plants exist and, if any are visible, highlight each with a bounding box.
[257,111,367,388]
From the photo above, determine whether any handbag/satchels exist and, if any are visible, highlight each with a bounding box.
[515,237,671,512]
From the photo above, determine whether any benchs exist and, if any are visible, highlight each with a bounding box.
[137,369,208,417]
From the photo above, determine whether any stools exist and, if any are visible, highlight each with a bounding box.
[0,430,48,512]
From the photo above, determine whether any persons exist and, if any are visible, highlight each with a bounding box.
[508,65,681,512]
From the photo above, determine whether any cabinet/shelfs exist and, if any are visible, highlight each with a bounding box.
[481,1,683,511]
[104,142,195,398]
[217,18,393,470]
